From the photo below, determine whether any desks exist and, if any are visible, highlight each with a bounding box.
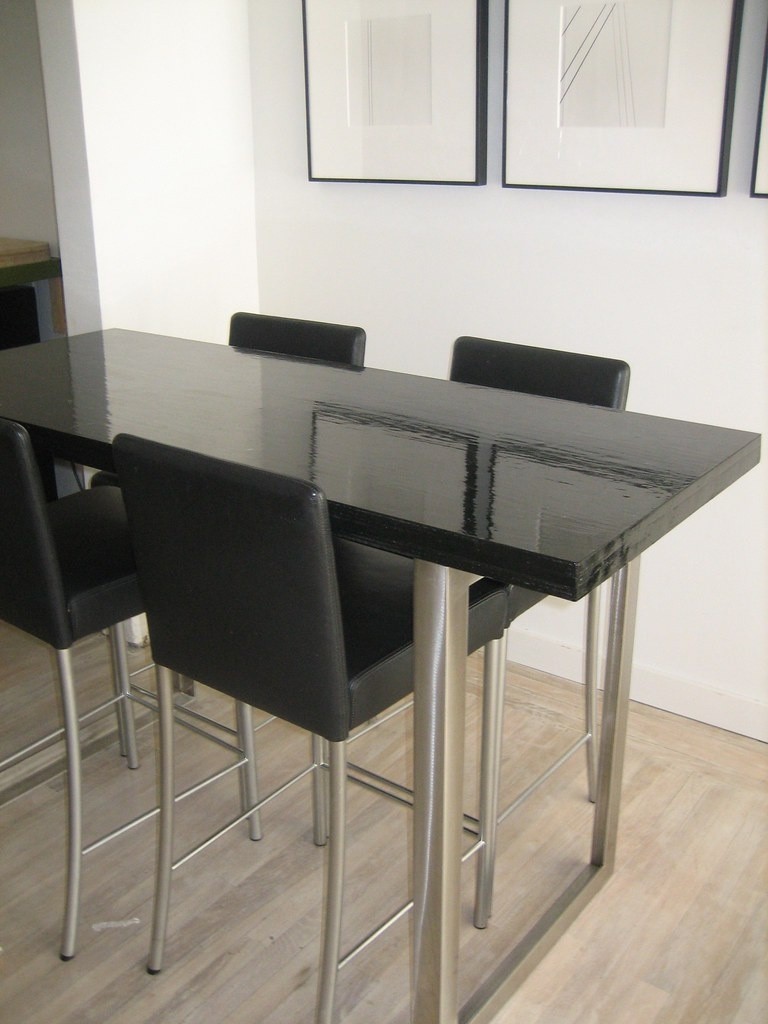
[0,327,762,1024]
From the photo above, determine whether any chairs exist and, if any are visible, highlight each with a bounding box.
[112,433,510,1024]
[322,336,631,922]
[92,311,366,813]
[0,418,263,964]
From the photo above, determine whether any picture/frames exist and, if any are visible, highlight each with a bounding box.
[300,0,489,187]
[749,25,768,199]
[502,0,745,198]
